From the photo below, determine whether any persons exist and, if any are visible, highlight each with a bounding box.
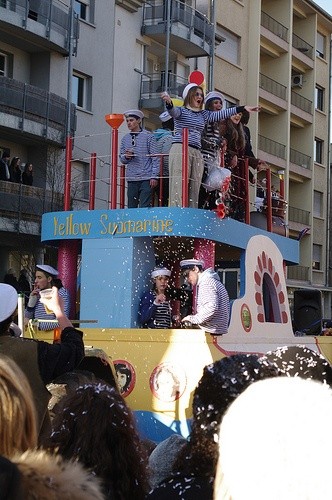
[0,152,33,186]
[25,265,71,331]
[0,283,332,500]
[138,270,171,329]
[256,177,280,213]
[180,259,230,334]
[4,268,35,309]
[119,84,262,223]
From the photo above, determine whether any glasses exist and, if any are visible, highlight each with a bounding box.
[131,135,136,146]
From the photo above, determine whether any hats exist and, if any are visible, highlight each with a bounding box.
[36,264,60,280]
[123,109,144,120]
[178,82,198,100]
[0,283,18,335]
[226,106,249,125]
[180,260,203,272]
[203,91,225,105]
[150,266,171,279]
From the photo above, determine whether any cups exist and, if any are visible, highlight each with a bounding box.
[39,289,53,314]
